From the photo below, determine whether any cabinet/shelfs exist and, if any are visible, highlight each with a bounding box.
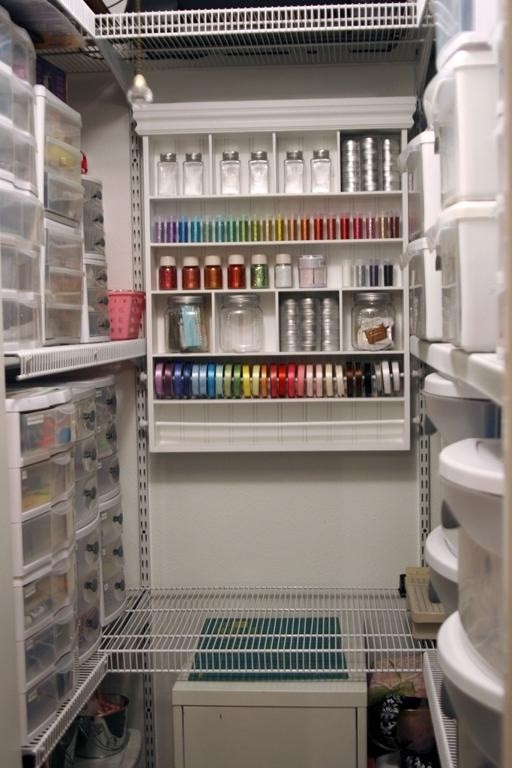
[350,291,399,353]
[308,148,332,190]
[180,152,205,194]
[284,148,306,193]
[219,149,242,194]
[155,152,179,197]
[216,294,267,352]
[0,0,146,768]
[158,253,295,291]
[164,293,212,353]
[246,149,270,195]
[402,326,510,767]
[127,97,415,453]
[169,621,372,768]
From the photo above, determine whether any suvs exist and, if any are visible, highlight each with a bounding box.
[66,692,132,759]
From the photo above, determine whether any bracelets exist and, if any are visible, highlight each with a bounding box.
[76,692,132,757]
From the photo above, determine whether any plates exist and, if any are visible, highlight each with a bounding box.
[127,97,415,453]
[402,326,510,767]
[169,621,372,768]
[0,0,146,768]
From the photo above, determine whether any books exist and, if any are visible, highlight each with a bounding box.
[0,19,116,349]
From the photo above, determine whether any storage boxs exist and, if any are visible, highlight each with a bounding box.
[394,2,509,354]
[6,387,79,746]
[0,19,116,349]
[87,378,125,628]
[71,384,102,665]
